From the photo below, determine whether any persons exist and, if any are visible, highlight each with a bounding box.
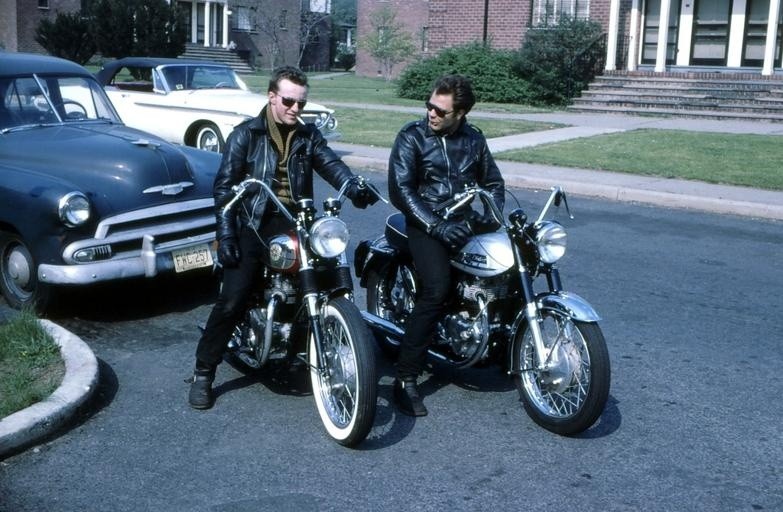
[188,65,381,410]
[388,77,504,416]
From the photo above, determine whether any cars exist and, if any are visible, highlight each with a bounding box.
[0,51,223,316]
[32,56,338,154]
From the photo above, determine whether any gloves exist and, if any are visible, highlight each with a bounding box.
[217,235,241,269]
[348,183,380,209]
[430,220,471,251]
[470,209,502,226]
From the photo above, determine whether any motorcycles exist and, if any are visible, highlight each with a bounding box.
[217,174,388,446]
[353,185,611,438]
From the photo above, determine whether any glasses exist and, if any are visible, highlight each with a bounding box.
[425,100,454,118]
[276,92,307,109]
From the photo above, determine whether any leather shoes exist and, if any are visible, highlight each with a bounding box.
[184,368,215,409]
[391,375,427,417]
